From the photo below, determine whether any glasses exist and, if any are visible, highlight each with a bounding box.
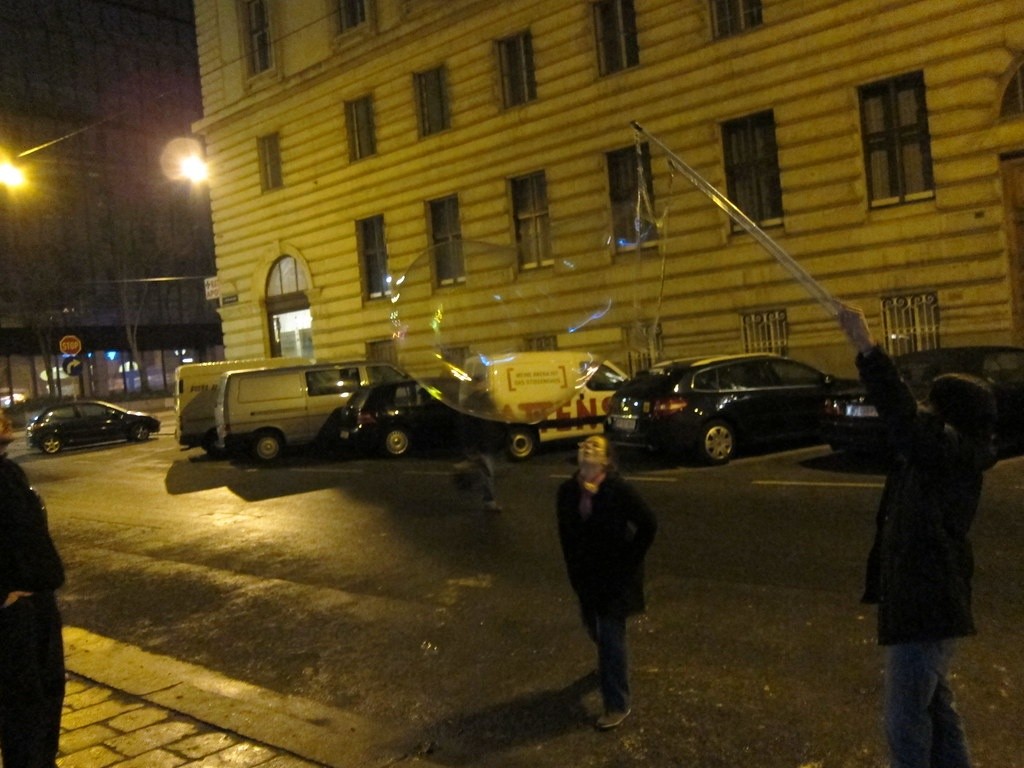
[577,440,608,452]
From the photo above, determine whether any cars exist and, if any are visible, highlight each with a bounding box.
[602,353,865,466]
[458,352,632,461]
[338,378,505,460]
[26,399,163,454]
[824,344,1022,468]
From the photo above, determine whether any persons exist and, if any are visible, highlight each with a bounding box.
[556,434,657,731]
[0,408,68,768]
[830,295,998,768]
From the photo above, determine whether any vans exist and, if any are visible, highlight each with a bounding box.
[173,355,341,459]
[212,358,422,465]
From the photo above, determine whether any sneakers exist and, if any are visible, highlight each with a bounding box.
[595,703,632,728]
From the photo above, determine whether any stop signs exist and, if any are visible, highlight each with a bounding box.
[60,335,82,356]
